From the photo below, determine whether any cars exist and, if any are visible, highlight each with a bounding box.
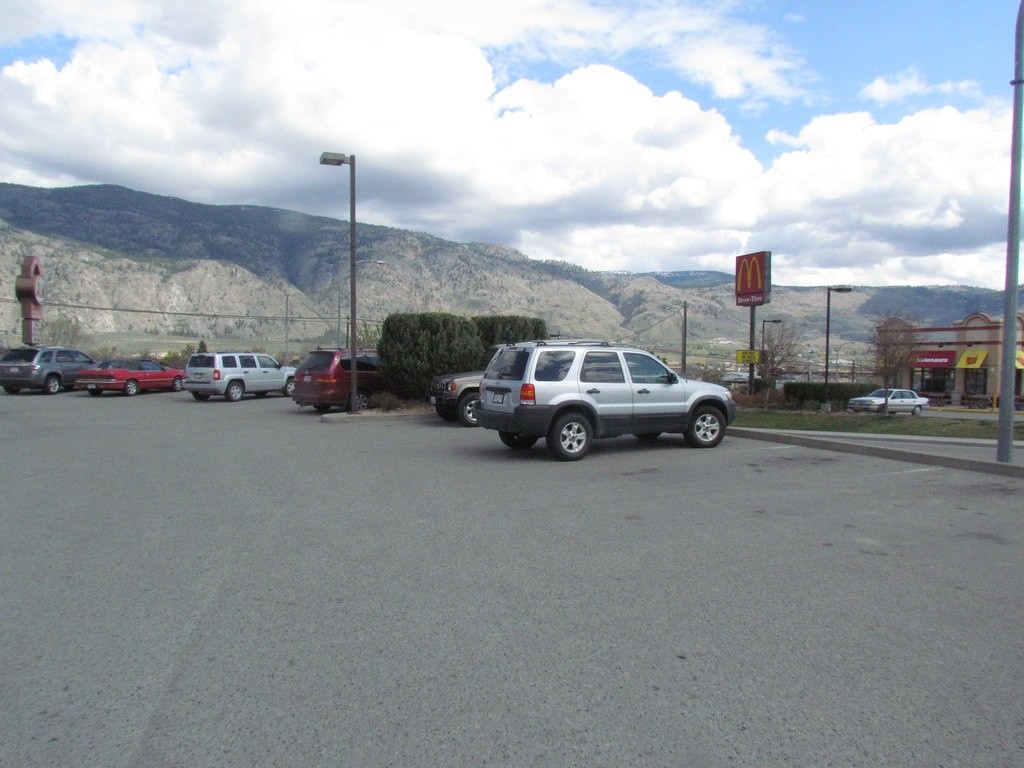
[846,387,931,416]
[0,345,113,395]
[74,357,186,396]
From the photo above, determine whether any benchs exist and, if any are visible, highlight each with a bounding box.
[937,399,950,401]
[1014,402,1024,405]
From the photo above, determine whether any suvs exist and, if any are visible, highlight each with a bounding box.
[292,346,391,413]
[182,351,298,403]
[474,336,737,463]
[432,338,648,428]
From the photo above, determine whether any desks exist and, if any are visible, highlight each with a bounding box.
[1014,396,1024,411]
[918,392,946,407]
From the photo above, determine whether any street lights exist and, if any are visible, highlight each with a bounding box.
[318,152,359,415]
[760,319,782,379]
[817,285,854,412]
[337,260,387,347]
